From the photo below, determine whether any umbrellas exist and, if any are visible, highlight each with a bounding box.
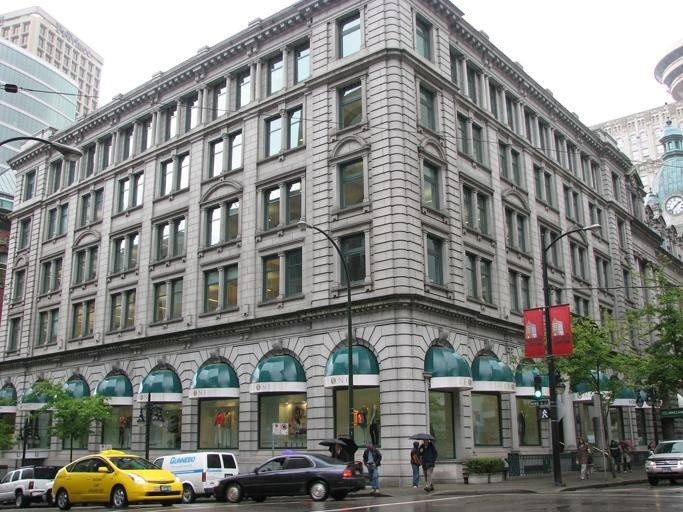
[410,433,438,449]
[319,436,359,455]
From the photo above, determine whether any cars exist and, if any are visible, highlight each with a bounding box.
[643,438,683,486]
[49,450,185,512]
[216,453,365,504]
[0,463,61,510]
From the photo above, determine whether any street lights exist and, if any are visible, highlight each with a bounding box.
[536,222,604,486]
[292,218,354,460]
[1,131,85,167]
[136,391,164,460]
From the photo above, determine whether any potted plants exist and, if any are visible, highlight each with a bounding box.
[464,456,510,485]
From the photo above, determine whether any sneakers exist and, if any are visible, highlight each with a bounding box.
[424,485,434,492]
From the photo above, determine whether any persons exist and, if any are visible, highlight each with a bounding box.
[352,401,379,446]
[576,438,591,481]
[362,440,382,495]
[214,410,232,448]
[333,444,350,462]
[118,415,133,448]
[167,413,178,448]
[609,438,635,474]
[647,440,656,455]
[410,439,438,492]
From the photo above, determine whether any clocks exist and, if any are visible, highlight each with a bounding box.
[664,195,683,217]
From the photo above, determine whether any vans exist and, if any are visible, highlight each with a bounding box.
[151,451,238,499]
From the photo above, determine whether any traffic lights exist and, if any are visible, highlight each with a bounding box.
[539,407,551,422]
[532,375,542,401]
[356,412,366,426]
